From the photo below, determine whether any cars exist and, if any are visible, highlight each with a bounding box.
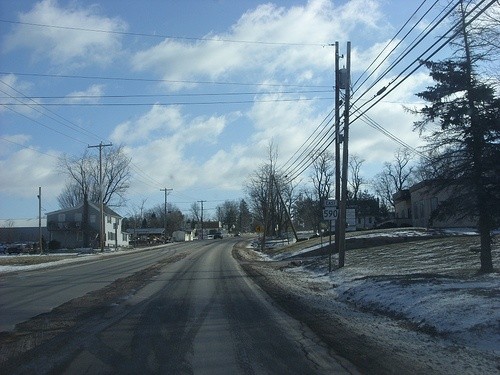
[129,236,174,245]
[8,244,33,255]
[234,233,241,237]
[323,231,335,237]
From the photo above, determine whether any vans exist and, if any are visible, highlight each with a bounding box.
[207,235,212,240]
[296,231,319,241]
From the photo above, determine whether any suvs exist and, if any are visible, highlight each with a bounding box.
[214,232,223,239]
